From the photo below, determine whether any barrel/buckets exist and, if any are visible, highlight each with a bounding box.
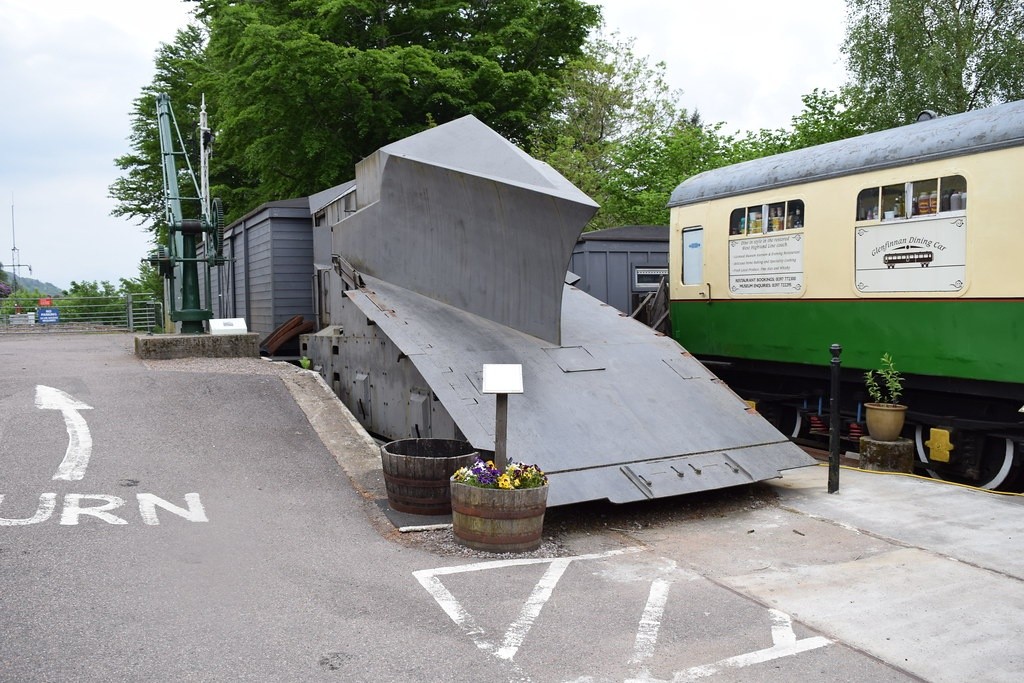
[380,439,480,514]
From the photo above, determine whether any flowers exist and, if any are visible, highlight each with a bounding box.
[452,457,549,490]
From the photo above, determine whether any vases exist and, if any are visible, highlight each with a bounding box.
[449,470,549,554]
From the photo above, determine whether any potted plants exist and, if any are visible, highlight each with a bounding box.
[864,352,909,442]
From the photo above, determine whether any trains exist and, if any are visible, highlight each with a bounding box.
[565,97,1024,494]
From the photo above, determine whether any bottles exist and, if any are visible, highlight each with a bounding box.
[894,189,966,217]
[740,209,802,234]
[867,205,879,220]
